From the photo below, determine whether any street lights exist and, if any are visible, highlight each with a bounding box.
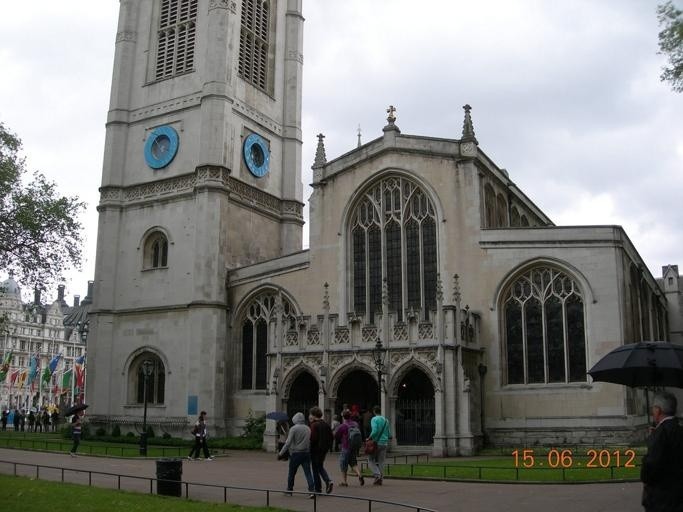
[370,338,387,415]
[139,357,154,457]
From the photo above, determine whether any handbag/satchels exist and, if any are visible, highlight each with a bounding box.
[365,441,376,453]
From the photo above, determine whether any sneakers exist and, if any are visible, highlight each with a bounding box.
[188,455,215,461]
[373,477,382,485]
[337,481,347,486]
[306,494,314,499]
[359,475,364,486]
[326,481,333,493]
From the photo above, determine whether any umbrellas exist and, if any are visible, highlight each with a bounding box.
[585,340,683,426]
[262,411,289,422]
[63,402,88,418]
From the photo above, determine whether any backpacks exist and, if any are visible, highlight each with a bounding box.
[348,427,362,450]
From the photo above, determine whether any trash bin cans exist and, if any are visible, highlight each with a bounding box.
[278,442,289,462]
[156,457,183,497]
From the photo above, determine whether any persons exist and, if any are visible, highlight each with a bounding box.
[187,418,212,462]
[639,393,683,512]
[194,410,214,460]
[0,410,60,432]
[68,411,81,457]
[276,404,391,499]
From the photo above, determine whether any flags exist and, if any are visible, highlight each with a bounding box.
[0,348,84,405]
